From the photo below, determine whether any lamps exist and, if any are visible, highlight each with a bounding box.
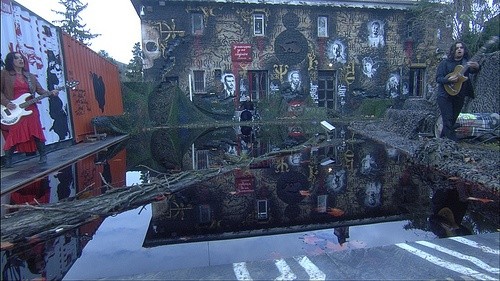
[140,5,146,15]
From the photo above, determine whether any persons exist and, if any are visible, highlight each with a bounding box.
[329,42,344,62]
[227,135,396,207]
[289,73,300,90]
[368,22,385,48]
[239,96,254,110]
[225,75,235,96]
[362,57,376,78]
[436,42,481,143]
[0,52,59,169]
[9,176,50,275]
[256,22,260,30]
[430,189,472,239]
[319,18,325,35]
[386,77,408,98]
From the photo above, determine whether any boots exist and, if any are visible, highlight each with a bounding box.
[5,147,14,168]
[34,138,47,164]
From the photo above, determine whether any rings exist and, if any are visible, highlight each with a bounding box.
[53,93,55,94]
